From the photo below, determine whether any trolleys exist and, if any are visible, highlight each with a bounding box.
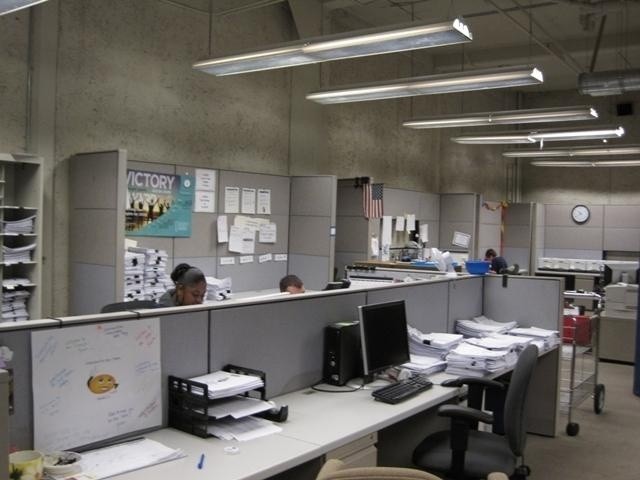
[560,288,607,439]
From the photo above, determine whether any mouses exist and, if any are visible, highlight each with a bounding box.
[441,378,463,388]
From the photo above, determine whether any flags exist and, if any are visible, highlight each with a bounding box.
[363,183,383,219]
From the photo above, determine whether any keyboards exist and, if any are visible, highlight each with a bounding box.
[371,375,433,406]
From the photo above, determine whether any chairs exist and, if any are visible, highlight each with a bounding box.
[410,342,538,480]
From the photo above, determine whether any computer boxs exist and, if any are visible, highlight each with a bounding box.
[323,321,361,386]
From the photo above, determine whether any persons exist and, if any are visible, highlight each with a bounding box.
[485,249,502,256]
[280,274,305,294]
[158,263,207,307]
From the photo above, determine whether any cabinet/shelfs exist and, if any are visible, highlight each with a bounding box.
[0,152,43,323]
[590,311,637,362]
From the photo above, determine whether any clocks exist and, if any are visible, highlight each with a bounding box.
[571,205,590,222]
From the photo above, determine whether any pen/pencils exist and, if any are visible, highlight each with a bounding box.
[199,454,206,468]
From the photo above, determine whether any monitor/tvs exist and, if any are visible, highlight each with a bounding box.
[325,282,350,289]
[358,299,410,375]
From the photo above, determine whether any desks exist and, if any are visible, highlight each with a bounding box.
[85,346,563,480]
